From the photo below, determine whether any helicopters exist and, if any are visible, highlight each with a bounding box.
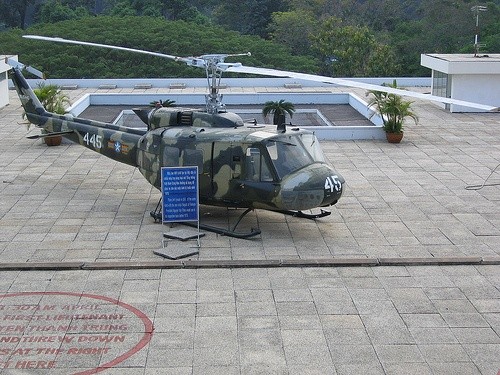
[4,34,499,239]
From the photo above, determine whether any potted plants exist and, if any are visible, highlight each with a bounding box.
[22,79,73,147]
[261,99,296,126]
[365,79,420,144]
